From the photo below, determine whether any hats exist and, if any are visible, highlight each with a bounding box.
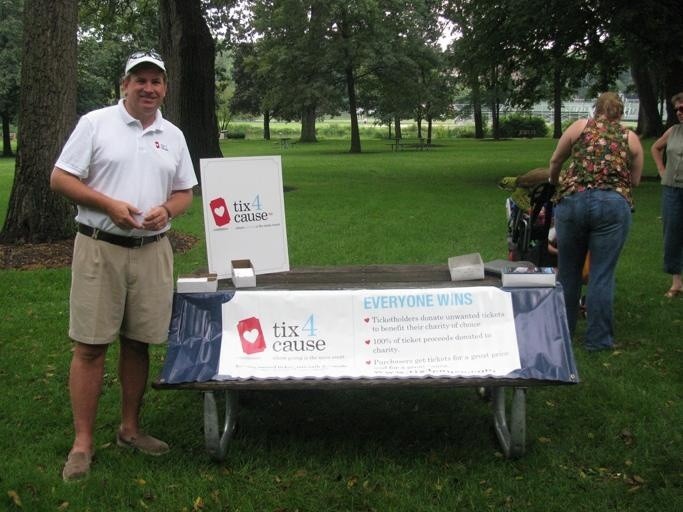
[125,52,168,81]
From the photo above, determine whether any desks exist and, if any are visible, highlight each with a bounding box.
[393,137,425,151]
[151,264,579,462]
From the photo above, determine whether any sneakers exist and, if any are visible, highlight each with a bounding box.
[116,426,170,457]
[62,444,96,483]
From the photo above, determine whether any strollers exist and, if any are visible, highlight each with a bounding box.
[498,170,588,321]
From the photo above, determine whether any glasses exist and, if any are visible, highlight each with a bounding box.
[673,104,683,114]
[127,51,168,66]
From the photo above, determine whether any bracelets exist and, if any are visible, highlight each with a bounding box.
[162,205,172,223]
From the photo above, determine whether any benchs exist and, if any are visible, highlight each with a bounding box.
[385,142,425,151]
[150,367,579,460]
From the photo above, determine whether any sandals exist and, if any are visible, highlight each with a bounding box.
[664,287,681,298]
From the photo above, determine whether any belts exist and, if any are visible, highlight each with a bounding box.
[78,222,167,249]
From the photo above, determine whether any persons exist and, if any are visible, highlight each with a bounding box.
[51,49,199,484]
[548,92,644,352]
[650,92,683,299]
